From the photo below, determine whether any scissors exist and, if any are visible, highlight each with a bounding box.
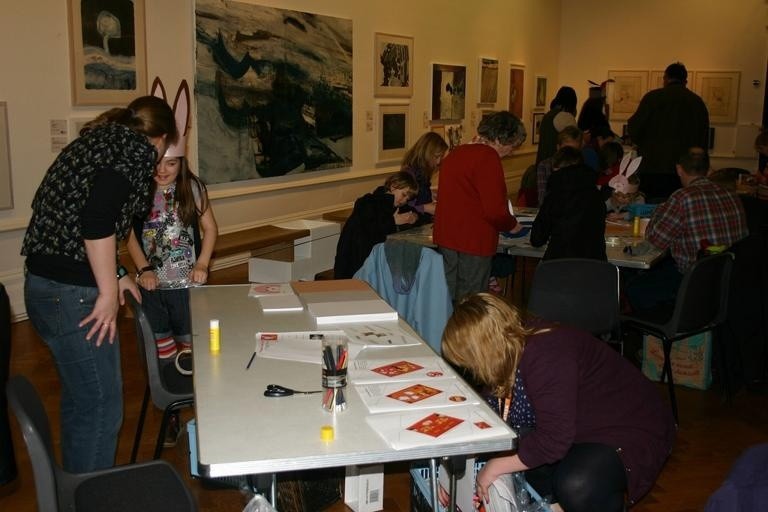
[263,383,321,397]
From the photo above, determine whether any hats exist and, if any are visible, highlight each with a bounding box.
[608,152,643,193]
[148,76,190,158]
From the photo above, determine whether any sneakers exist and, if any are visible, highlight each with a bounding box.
[489,276,501,293]
[161,417,184,448]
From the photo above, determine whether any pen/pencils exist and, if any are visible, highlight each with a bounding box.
[320,345,347,412]
[245,351,256,369]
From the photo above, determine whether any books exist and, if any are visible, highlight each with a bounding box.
[259,294,304,312]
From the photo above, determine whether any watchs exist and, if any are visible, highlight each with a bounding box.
[117,265,129,280]
[135,265,154,277]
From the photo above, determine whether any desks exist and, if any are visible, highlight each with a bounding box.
[118,288,202,467]
[189,279,518,512]
[387,207,672,353]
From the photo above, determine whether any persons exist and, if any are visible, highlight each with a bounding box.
[530,145,608,264]
[436,290,677,512]
[627,62,710,205]
[123,75,219,448]
[535,86,586,166]
[644,146,750,326]
[606,172,645,222]
[432,109,528,306]
[736,132,767,199]
[577,85,624,190]
[400,131,449,215]
[19,94,177,474]
[536,124,584,204]
[332,170,420,280]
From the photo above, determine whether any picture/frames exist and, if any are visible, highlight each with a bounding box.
[534,74,548,111]
[695,70,742,125]
[606,69,650,122]
[190,0,357,187]
[651,69,694,93]
[478,55,499,105]
[374,101,411,168]
[506,62,526,122]
[430,62,470,123]
[69,2,148,108]
[533,113,545,145]
[372,31,414,98]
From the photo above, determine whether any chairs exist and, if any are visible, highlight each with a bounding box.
[4,373,197,512]
[615,254,733,426]
[722,233,766,399]
[529,258,626,358]
